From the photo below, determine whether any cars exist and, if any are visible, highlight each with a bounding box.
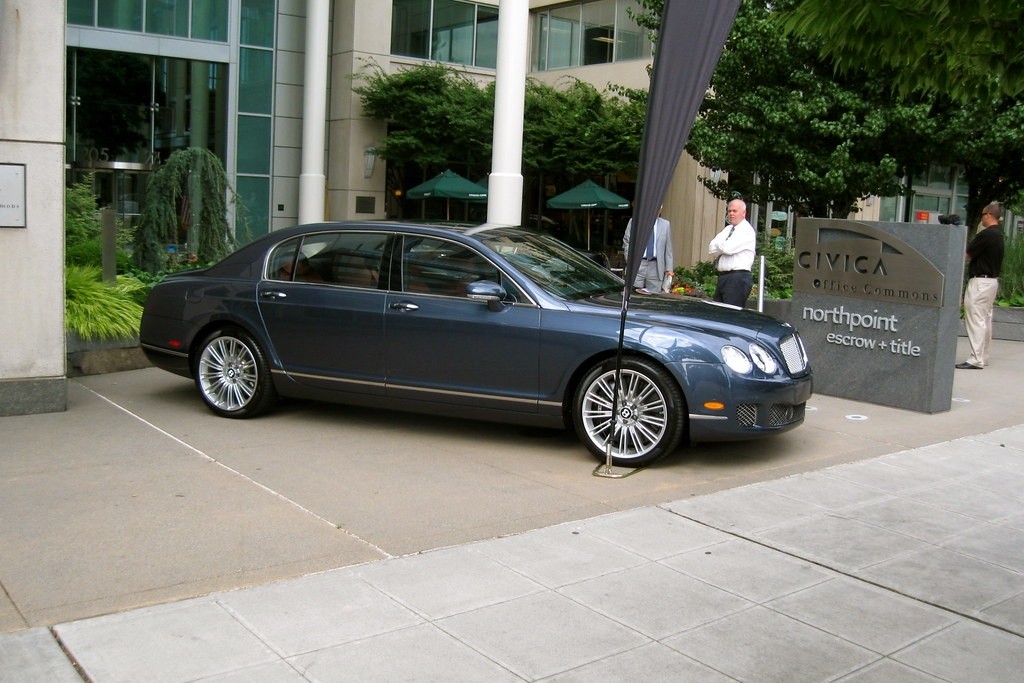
[140,220,814,469]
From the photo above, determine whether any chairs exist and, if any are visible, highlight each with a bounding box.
[336,256,376,287]
[451,257,474,297]
[408,260,430,293]
[283,253,328,283]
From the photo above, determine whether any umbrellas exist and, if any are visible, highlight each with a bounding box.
[546,181,630,253]
[408,169,488,221]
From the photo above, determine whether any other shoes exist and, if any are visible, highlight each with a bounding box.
[955,362,983,369]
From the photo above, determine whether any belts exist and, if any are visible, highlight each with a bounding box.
[715,270,747,276]
[642,257,657,261]
[970,275,996,278]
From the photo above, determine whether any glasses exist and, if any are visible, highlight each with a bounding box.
[979,212,987,217]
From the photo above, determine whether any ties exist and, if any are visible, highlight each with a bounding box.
[645,227,655,260]
[715,225,736,264]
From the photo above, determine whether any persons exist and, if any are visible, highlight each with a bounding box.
[955,203,1004,370]
[623,199,674,292]
[708,199,755,308]
